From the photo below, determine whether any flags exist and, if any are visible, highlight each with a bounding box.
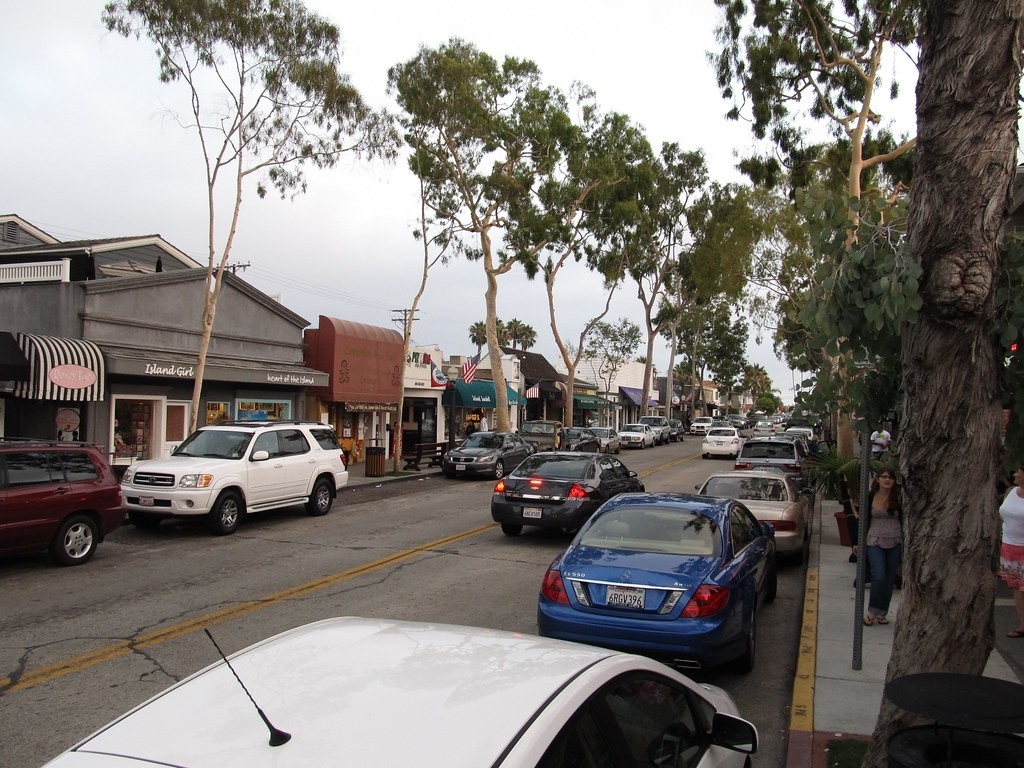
[461,354,480,385]
[430,360,450,387]
[525,383,538,398]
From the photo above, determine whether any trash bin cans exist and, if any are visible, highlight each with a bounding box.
[365,446,386,478]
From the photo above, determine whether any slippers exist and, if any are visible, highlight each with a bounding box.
[877,616,889,624]
[863,614,873,626]
[1006,629,1024,638]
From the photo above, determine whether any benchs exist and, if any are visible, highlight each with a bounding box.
[401,440,462,471]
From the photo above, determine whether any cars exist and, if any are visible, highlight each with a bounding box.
[689,416,713,435]
[700,427,748,460]
[515,419,567,454]
[735,433,809,472]
[767,412,793,425]
[442,432,535,480]
[536,492,778,677]
[727,414,767,430]
[637,416,671,446]
[707,421,734,434]
[617,424,656,449]
[785,418,811,431]
[40,616,759,768]
[558,427,601,453]
[785,426,820,444]
[587,427,623,454]
[666,418,685,442]
[712,416,728,422]
[753,421,777,437]
[743,464,790,475]
[491,452,647,537]
[694,470,815,564]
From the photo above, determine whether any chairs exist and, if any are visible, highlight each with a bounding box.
[531,427,541,432]
[713,483,735,496]
[753,448,764,452]
[548,428,560,448]
[601,521,629,540]
[681,540,706,547]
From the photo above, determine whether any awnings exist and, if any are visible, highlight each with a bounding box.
[442,379,527,408]
[619,386,658,407]
[11,332,105,402]
[0,331,31,381]
[557,394,612,409]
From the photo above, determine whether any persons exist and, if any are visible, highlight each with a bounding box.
[848,467,904,626]
[998,465,1024,638]
[465,419,475,436]
[478,413,488,432]
[869,423,892,460]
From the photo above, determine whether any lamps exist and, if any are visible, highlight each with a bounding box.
[416,343,440,352]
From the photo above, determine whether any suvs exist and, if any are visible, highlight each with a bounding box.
[121,419,348,535]
[0,435,127,567]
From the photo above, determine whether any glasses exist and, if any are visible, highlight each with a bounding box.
[877,475,894,479]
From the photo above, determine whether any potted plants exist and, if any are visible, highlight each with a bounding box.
[801,443,883,547]
